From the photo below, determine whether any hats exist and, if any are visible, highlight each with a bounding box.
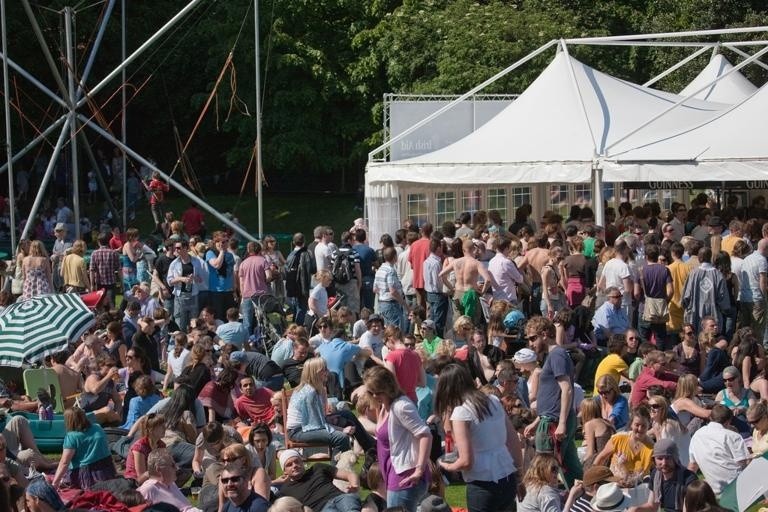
[583,465,630,512]
[514,348,538,363]
[55,223,68,231]
[230,351,247,362]
[708,217,725,226]
[279,449,302,472]
[368,314,383,321]
[422,319,436,329]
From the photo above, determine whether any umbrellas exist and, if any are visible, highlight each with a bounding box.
[0,293,97,369]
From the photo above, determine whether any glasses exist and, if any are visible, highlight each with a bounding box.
[126,355,136,359]
[175,246,183,250]
[552,466,559,473]
[665,229,674,233]
[748,417,762,425]
[218,240,229,243]
[222,455,242,464]
[368,390,385,396]
[554,255,565,262]
[420,326,429,331]
[629,337,640,341]
[526,335,543,341]
[685,332,696,336]
[723,377,736,382]
[221,476,245,484]
[678,209,687,212]
[635,231,644,236]
[318,325,329,329]
[597,387,614,397]
[647,404,662,410]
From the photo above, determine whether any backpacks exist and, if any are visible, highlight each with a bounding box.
[332,247,354,284]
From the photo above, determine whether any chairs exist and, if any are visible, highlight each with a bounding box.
[67,392,83,410]
[280,386,334,463]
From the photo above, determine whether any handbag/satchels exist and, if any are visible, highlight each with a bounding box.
[642,297,669,325]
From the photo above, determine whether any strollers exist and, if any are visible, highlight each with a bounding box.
[250,290,319,356]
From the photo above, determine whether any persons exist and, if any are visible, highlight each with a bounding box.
[52,151,66,182]
[16,168,30,201]
[49,321,127,424]
[0,376,56,417]
[0,414,59,471]
[140,157,171,232]
[24,476,75,511]
[120,193,768,511]
[89,146,139,220]
[0,433,31,512]
[50,406,115,492]
[0,196,89,294]
[89,220,120,313]
